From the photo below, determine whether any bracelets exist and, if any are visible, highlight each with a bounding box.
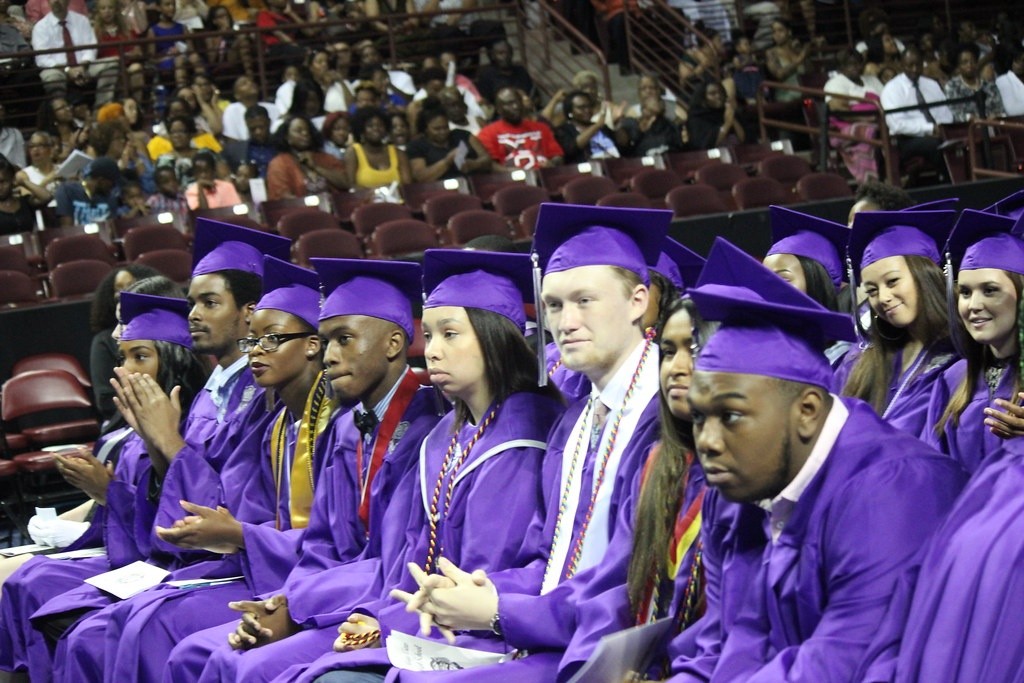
[600,111,606,116]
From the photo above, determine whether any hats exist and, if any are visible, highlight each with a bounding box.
[646,237,707,293]
[251,254,320,329]
[844,210,955,270]
[763,205,859,323]
[119,292,195,351]
[310,257,424,344]
[422,249,547,387]
[686,236,857,393]
[190,216,293,282]
[532,202,674,289]
[938,208,1024,274]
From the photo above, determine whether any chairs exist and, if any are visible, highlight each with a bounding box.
[1,72,1024,533]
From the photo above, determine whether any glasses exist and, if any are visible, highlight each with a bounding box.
[237,332,317,352]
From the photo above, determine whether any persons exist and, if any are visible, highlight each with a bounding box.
[0,182,1024,683]
[591,0,1024,190]
[0,0,744,238]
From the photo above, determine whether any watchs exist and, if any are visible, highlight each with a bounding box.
[490,614,503,636]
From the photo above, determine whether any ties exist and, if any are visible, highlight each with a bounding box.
[912,81,935,123]
